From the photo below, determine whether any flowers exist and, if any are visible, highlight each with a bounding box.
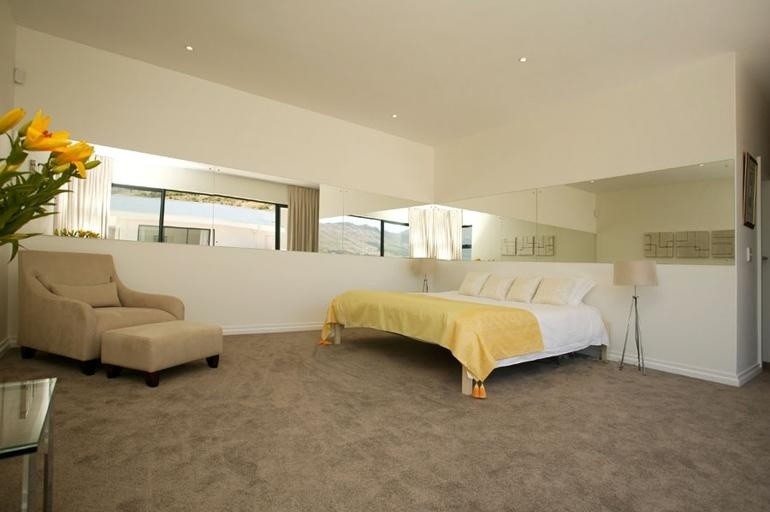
[0,107,103,264]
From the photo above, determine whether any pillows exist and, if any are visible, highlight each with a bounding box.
[49,282,121,310]
[457,271,598,307]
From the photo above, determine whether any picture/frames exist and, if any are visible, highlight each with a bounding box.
[743,152,758,230]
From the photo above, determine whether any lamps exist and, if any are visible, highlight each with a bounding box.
[611,260,659,376]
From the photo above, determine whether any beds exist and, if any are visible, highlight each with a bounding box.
[321,270,608,395]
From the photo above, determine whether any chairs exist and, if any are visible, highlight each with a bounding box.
[16,248,185,375]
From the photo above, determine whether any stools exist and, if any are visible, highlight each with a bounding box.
[100,316,225,387]
